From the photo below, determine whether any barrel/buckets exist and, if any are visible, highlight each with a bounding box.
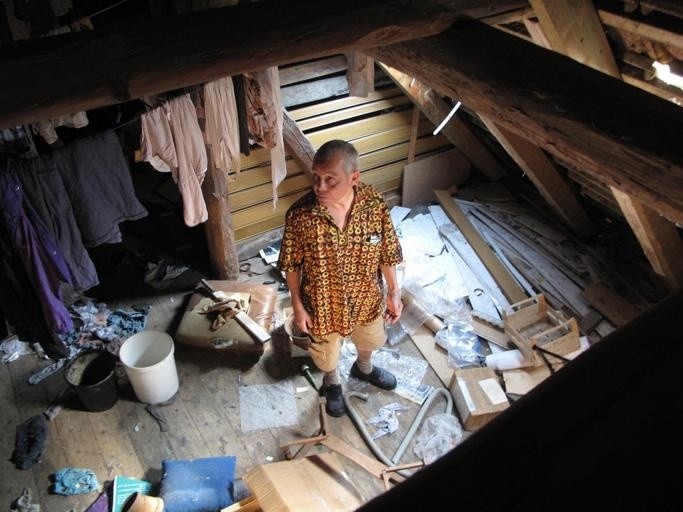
[118,330,180,405]
[63,349,118,412]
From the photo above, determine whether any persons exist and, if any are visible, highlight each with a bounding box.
[277,139,404,418]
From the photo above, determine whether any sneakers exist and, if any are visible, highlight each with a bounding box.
[351,362,396,390]
[323,377,345,417]
